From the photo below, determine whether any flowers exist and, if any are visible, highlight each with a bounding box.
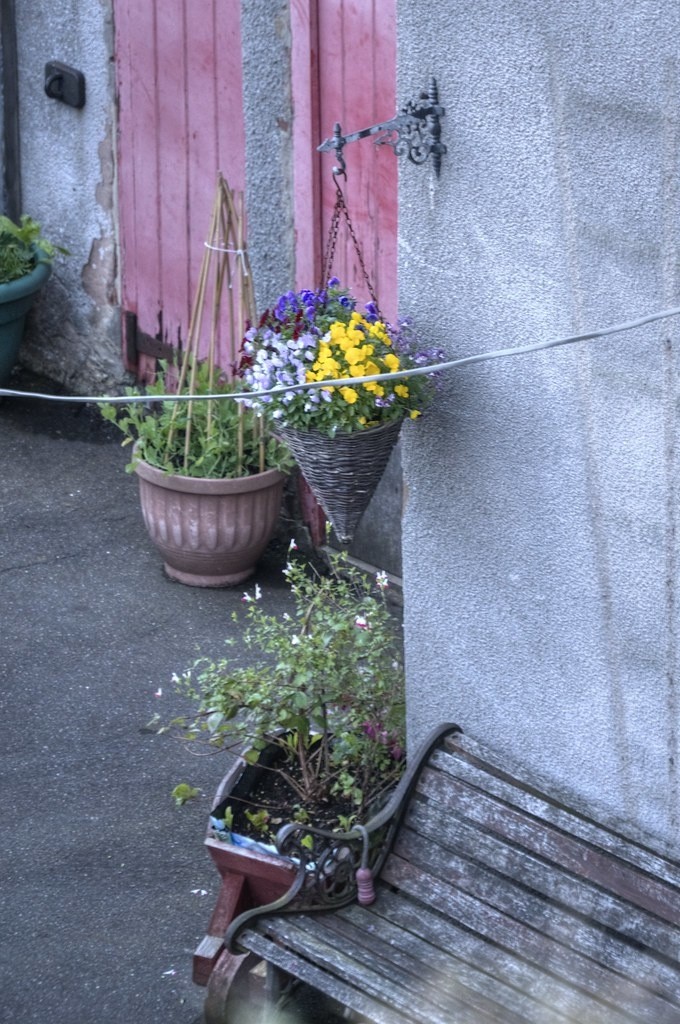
[231,275,436,438]
[131,518,405,853]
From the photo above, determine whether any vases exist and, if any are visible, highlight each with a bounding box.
[190,727,386,987]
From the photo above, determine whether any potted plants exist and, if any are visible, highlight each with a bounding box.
[96,351,294,588]
[0,216,72,387]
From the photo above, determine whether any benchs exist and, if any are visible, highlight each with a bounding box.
[202,723,680,1024]
